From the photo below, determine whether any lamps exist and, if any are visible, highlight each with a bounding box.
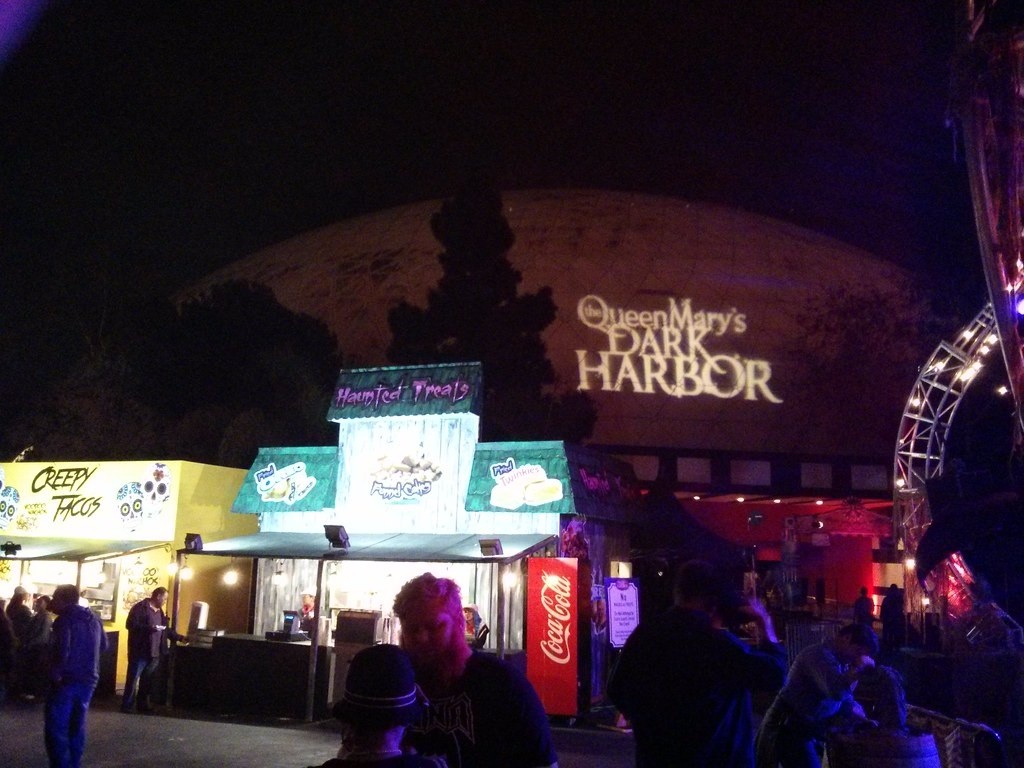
[0,541,23,556]
[183,533,202,551]
[479,539,503,556]
[323,524,350,553]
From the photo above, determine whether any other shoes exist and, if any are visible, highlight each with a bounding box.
[20,693,35,700]
[120,708,133,713]
[138,709,158,715]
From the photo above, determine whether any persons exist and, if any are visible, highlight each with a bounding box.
[308,644,447,768]
[393,572,558,768]
[611,552,1024,768]
[118,586,196,713]
[297,585,320,620]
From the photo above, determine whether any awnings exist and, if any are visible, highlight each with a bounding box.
[0,584,110,768]
[164,531,560,721]
[0,535,176,707]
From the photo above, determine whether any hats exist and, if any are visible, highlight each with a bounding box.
[331,644,426,727]
[14,586,28,599]
